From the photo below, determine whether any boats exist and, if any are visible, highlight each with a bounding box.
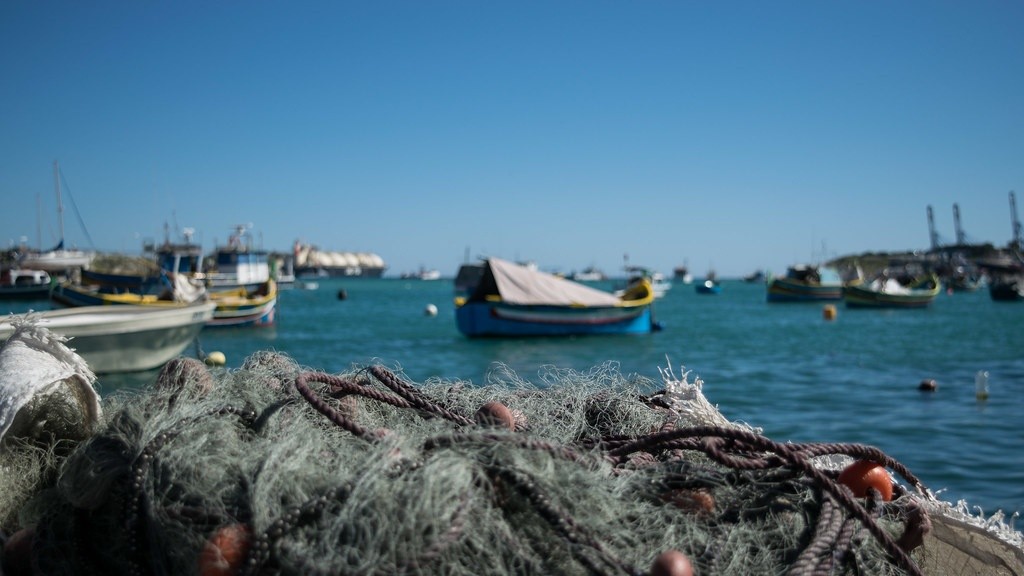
[398,242,768,295]
[453,251,666,338]
[765,263,865,301]
[920,187,1023,300]
[848,263,942,307]
[0,148,387,376]
